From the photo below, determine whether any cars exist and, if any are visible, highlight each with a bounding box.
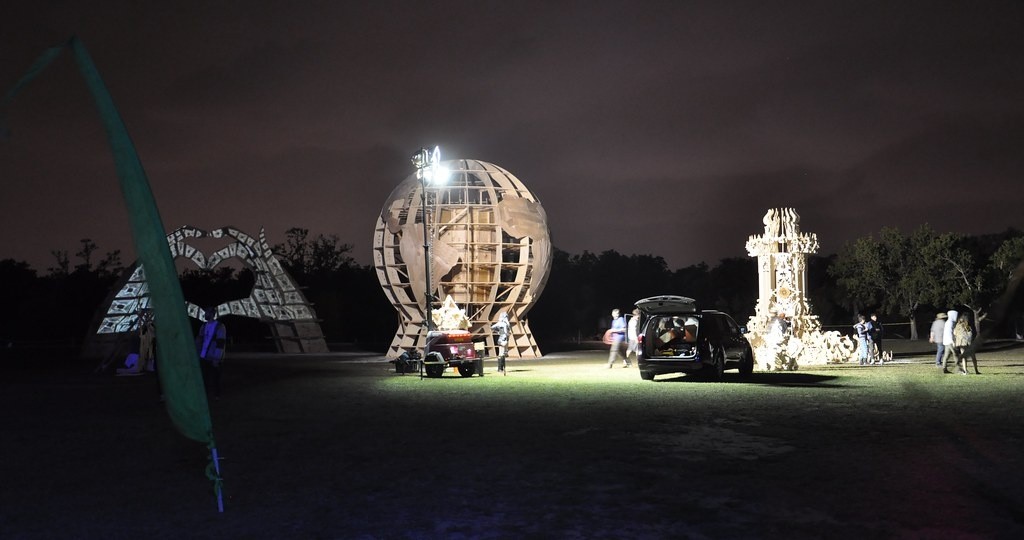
[634,294,753,383]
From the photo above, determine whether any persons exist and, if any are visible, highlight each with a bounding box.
[856,310,982,376]
[607,308,638,370]
[197,308,228,400]
[765,313,798,371]
[492,312,511,375]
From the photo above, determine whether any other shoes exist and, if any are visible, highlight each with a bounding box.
[944,371,952,373]
[975,371,982,374]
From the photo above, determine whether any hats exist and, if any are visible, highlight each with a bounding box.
[936,312,948,319]
